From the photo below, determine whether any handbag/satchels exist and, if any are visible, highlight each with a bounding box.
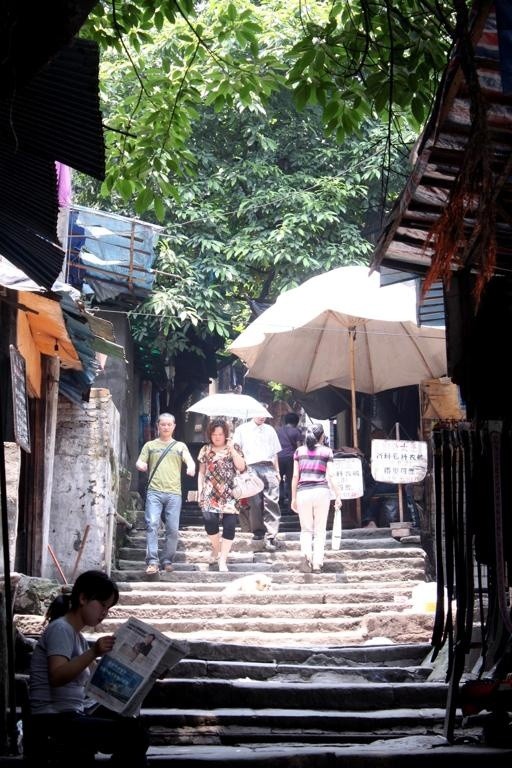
[142,480,148,511]
[232,471,264,500]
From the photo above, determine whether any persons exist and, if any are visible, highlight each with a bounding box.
[291,423,342,570]
[276,414,303,514]
[47,595,72,620]
[196,419,245,573]
[354,429,389,527]
[133,634,155,656]
[27,569,151,767]
[136,413,196,575]
[235,402,282,550]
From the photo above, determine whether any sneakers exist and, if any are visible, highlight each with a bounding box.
[263,538,276,551]
[145,563,159,576]
[218,557,229,572]
[303,554,312,572]
[163,564,173,572]
[253,531,264,540]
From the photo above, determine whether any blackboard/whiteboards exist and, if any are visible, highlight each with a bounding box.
[326,458,365,500]
[371,439,428,484]
[9,344,31,454]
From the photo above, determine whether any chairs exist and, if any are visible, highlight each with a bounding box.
[11,672,95,767]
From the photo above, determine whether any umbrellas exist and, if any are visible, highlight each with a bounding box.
[186,393,273,421]
[225,266,447,528]
